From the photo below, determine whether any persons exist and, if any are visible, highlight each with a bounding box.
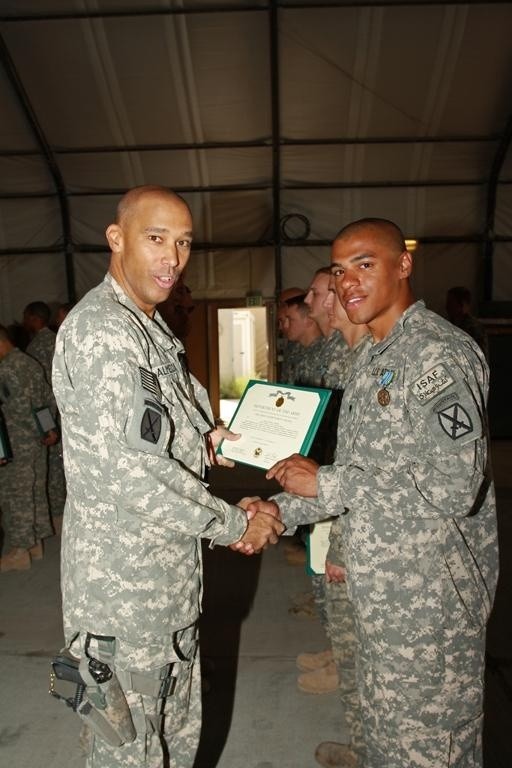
[232,221,498,767]
[52,185,286,768]
[272,267,374,691]
[0,301,71,573]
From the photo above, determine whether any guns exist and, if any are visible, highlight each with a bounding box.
[50,655,112,712]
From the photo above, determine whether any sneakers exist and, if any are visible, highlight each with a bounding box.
[314,740,359,768]
[0,542,43,571]
[296,650,340,694]
[283,545,305,565]
[52,515,63,536]
[288,591,318,620]
